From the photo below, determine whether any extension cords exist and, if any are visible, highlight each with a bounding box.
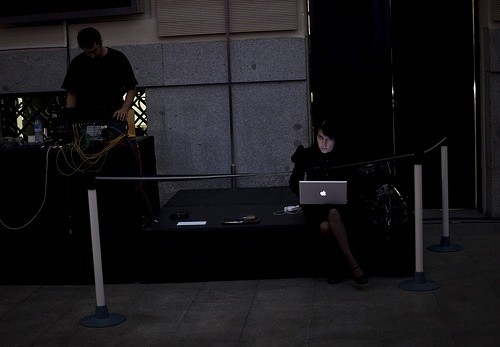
[284,205,299,212]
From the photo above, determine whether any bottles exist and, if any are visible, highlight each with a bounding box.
[34,116,44,145]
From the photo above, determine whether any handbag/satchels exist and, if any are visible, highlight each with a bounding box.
[375,184,409,224]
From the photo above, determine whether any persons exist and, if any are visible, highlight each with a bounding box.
[62,27,138,121]
[289,118,368,284]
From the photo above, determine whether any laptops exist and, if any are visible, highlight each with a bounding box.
[299,180,347,205]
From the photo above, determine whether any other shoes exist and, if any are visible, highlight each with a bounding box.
[347,264,368,284]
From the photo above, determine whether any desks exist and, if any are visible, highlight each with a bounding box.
[0,136,161,284]
[146,187,307,231]
